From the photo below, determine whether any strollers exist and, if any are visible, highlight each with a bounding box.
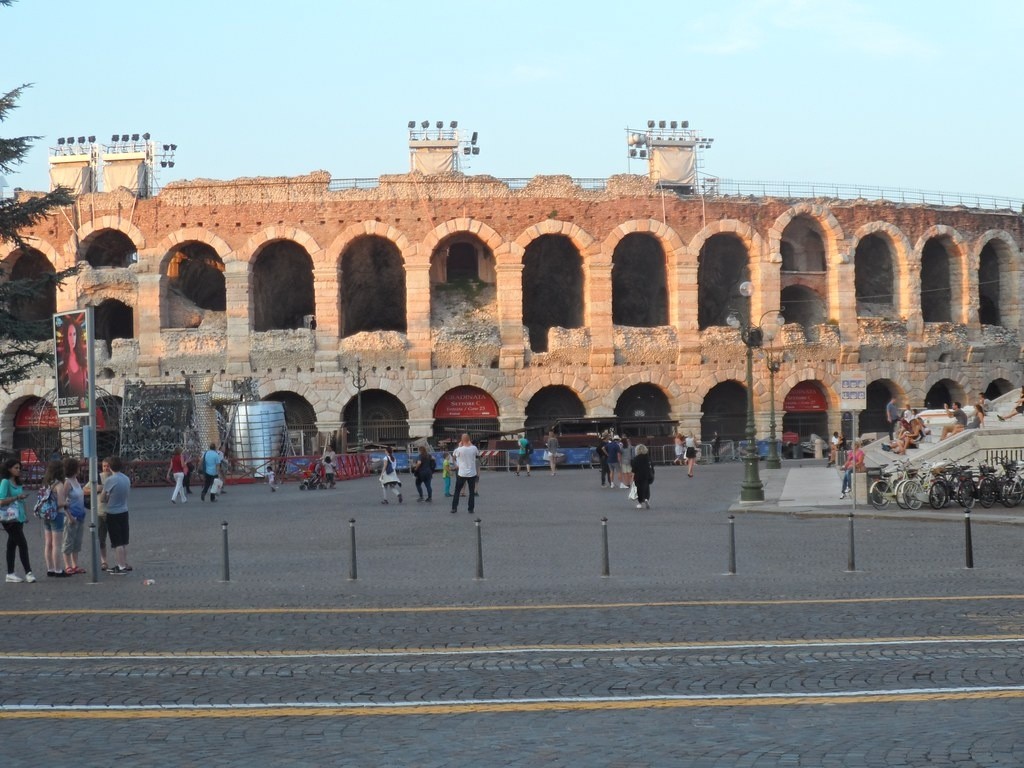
[299,458,328,490]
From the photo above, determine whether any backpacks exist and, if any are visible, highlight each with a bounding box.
[526,440,534,454]
[198,451,207,473]
[84,475,102,509]
[32,482,61,520]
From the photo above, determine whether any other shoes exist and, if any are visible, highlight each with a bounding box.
[635,504,642,509]
[513,472,521,477]
[381,492,480,517]
[643,499,650,510]
[601,483,629,488]
[997,415,1005,421]
[526,472,530,477]
[170,489,226,503]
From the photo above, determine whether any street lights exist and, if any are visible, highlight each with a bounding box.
[755,332,792,472]
[341,353,380,455]
[726,281,787,501]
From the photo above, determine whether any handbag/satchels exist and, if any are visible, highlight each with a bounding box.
[628,482,638,500]
[386,457,393,474]
[0,501,20,521]
[210,478,223,494]
[646,462,654,484]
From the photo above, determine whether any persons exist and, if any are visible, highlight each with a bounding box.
[996,385,1024,420]
[514,435,530,476]
[57,318,88,398]
[712,430,720,463]
[380,445,403,504]
[827,432,846,467]
[881,392,992,455]
[414,446,435,503]
[547,431,559,476]
[41,456,132,577]
[840,442,865,499]
[596,433,653,509]
[310,316,316,330]
[315,445,338,489]
[443,433,480,512]
[167,443,229,503]
[264,467,276,491]
[673,431,701,477]
[0,458,36,583]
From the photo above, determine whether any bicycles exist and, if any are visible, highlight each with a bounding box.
[868,455,1024,510]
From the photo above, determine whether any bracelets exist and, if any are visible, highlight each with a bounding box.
[16,495,19,500]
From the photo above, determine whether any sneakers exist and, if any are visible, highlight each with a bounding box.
[6,562,132,582]
[845,488,851,493]
[841,493,847,499]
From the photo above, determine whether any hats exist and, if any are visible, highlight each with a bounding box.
[601,433,609,440]
[612,434,621,440]
